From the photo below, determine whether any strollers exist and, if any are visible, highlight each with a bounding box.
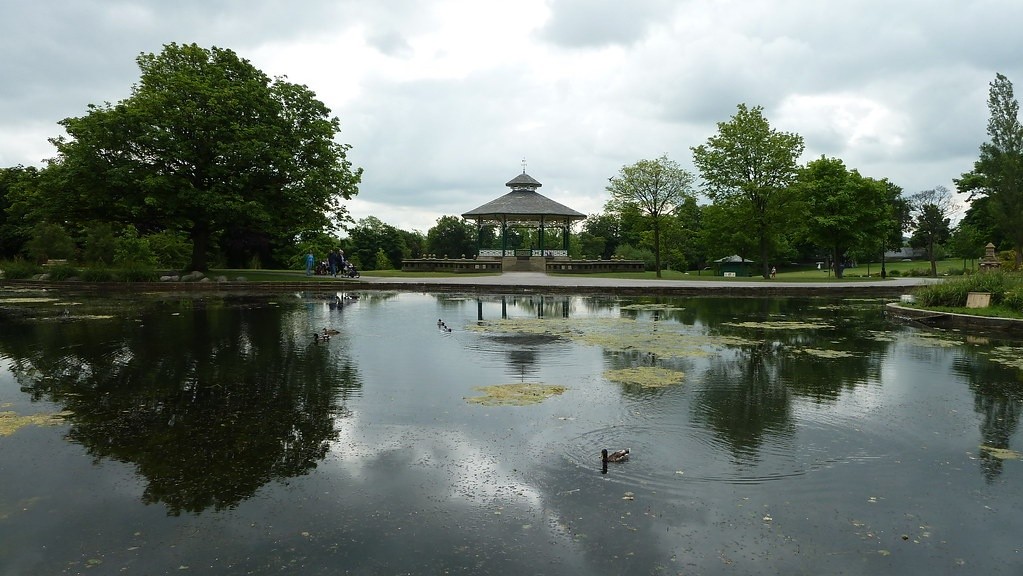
[347,263,360,278]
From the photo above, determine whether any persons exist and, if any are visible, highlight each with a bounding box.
[772,266,776,277]
[307,249,351,277]
